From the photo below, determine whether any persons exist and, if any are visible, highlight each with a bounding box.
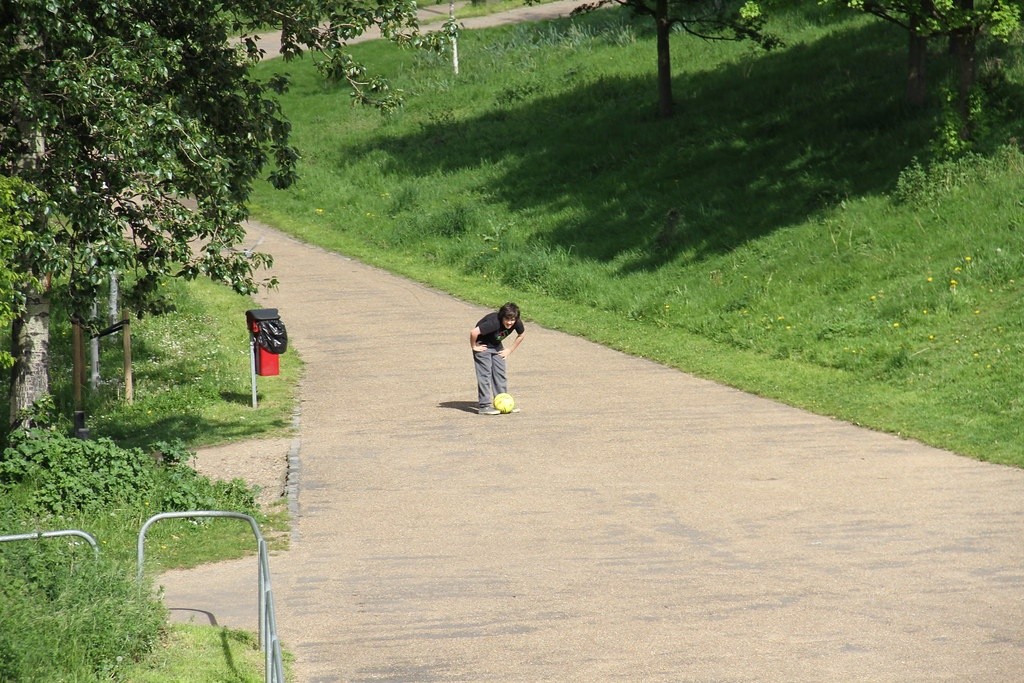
[470,303,525,415]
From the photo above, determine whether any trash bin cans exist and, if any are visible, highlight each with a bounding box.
[245,307,281,376]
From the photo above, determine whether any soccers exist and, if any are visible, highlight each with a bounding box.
[493,392,515,414]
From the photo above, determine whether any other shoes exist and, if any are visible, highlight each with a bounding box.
[512,408,519,412]
[478,407,500,414]
